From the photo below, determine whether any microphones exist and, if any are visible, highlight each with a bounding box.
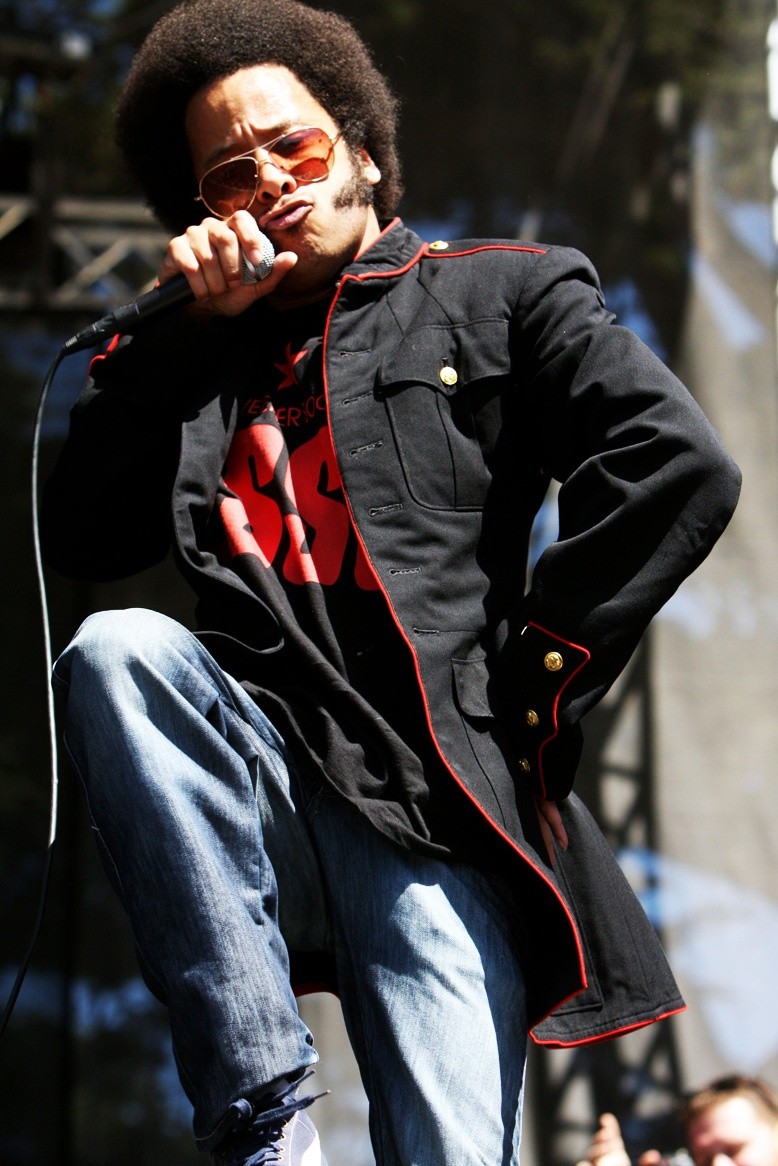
[60,231,274,355]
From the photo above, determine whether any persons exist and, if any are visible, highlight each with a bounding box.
[51,0,743,1166]
[576,1071,778,1166]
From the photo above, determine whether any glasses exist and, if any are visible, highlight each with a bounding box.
[194,131,346,220]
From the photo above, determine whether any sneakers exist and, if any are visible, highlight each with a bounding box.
[189,1070,322,1166]
